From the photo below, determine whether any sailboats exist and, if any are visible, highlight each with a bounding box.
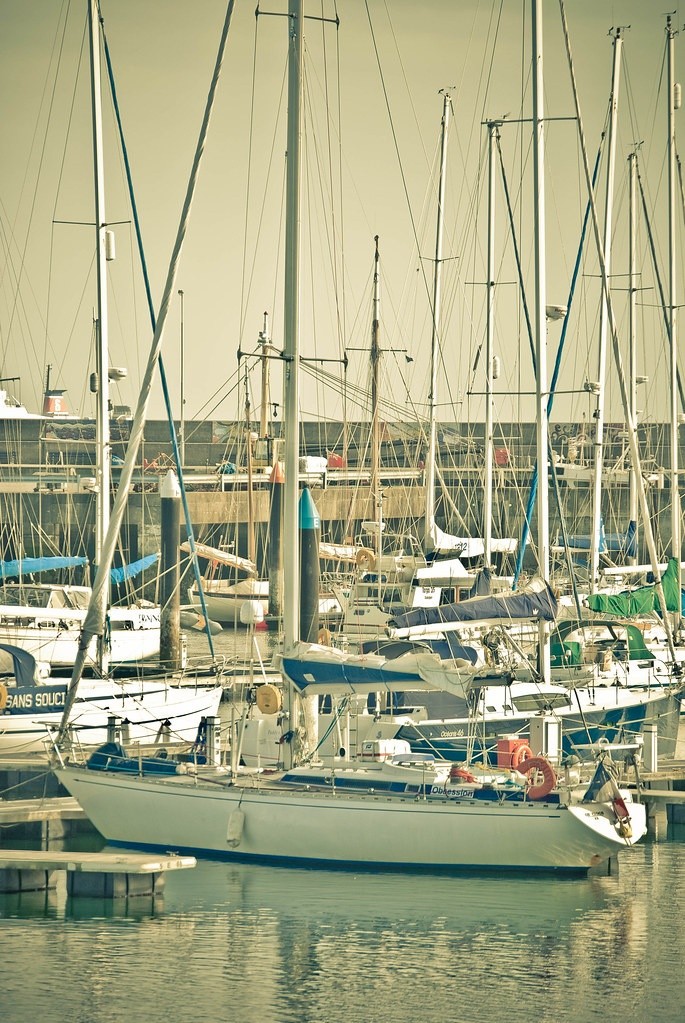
[0,0,685,880]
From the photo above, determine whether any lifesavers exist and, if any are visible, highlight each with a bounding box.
[356,549,377,572]
[195,575,206,591]
[515,758,557,799]
[0,684,7,710]
[317,627,331,646]
[512,745,535,779]
